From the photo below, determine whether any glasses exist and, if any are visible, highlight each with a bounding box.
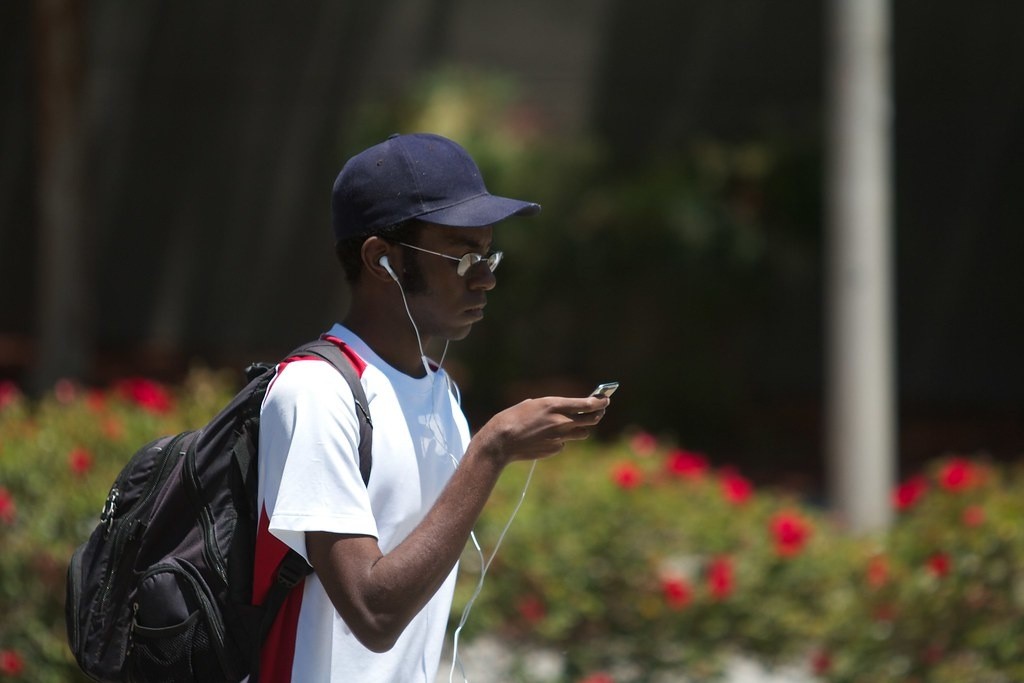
[397,238,505,279]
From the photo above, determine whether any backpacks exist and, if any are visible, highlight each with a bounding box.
[65,339,373,683]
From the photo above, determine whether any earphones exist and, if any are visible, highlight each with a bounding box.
[379,256,398,283]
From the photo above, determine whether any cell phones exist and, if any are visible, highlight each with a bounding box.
[580,383,620,415]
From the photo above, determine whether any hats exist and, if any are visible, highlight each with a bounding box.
[330,134,544,247]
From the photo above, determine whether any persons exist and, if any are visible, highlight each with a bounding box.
[247,133,610,683]
